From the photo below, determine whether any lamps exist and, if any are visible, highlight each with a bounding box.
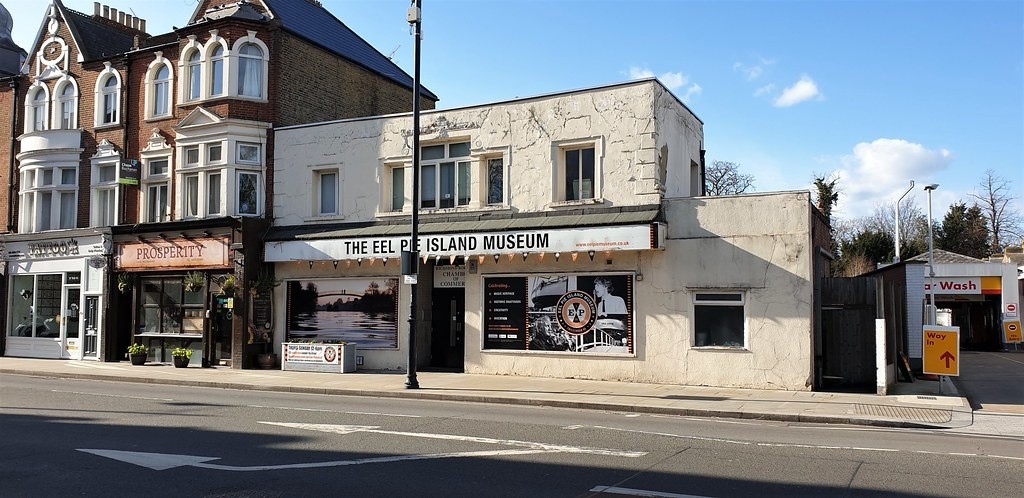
[134,230,210,242]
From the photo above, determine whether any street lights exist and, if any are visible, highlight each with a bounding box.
[924,181,941,325]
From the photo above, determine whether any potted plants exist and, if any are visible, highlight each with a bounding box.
[183,271,204,292]
[255,352,277,370]
[171,348,192,368]
[127,342,149,364]
[220,276,239,296]
[118,272,133,292]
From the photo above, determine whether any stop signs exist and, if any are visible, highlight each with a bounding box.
[1008,305,1015,311]
[1008,324,1017,332]
